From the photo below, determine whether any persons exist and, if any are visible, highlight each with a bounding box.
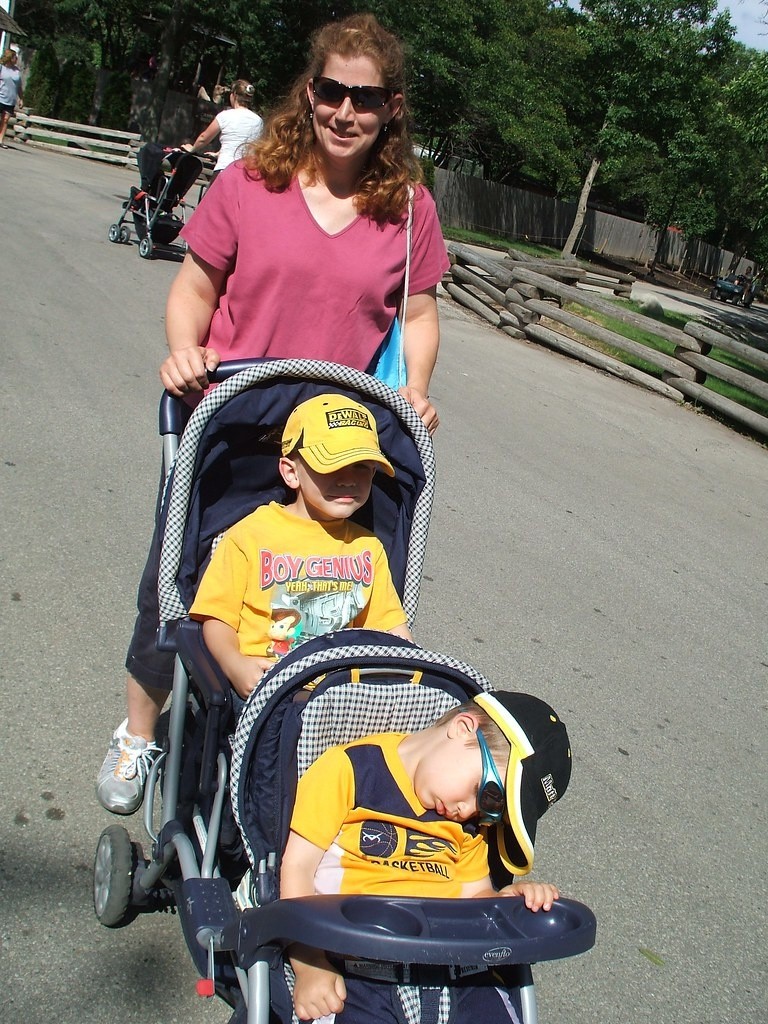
[275,689,572,1024]
[188,394,413,732]
[0,50,22,148]
[94,16,450,814]
[734,266,755,301]
[181,79,264,203]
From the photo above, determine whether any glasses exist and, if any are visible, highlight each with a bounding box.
[311,75,401,111]
[458,707,506,830]
[11,56,18,60]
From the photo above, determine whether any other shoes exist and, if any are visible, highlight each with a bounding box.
[0,143,8,149]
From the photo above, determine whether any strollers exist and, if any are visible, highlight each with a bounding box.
[90,362,598,1024]
[105,143,217,260]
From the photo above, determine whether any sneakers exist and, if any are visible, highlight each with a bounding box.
[94,717,162,814]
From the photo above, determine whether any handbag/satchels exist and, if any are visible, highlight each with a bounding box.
[363,180,415,395]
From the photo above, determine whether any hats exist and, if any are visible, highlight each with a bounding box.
[472,690,573,875]
[282,393,395,478]
